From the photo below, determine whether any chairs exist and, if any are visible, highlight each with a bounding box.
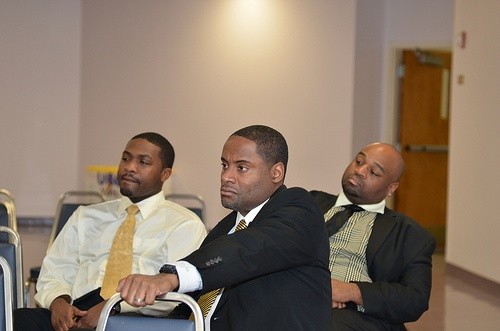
[0,190,207,331]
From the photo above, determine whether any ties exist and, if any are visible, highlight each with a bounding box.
[325,205,365,237]
[99,204,140,300]
[189,220,247,322]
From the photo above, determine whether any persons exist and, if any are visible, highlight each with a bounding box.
[310,142,436,331]
[14,132,207,331]
[117,124,333,331]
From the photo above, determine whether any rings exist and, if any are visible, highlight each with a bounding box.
[133,298,144,302]
